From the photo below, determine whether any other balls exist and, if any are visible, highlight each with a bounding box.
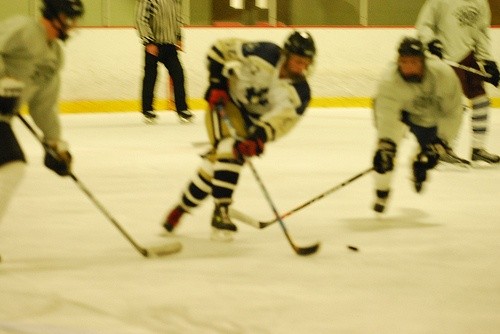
[348,245,358,252]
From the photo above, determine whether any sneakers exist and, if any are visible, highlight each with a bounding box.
[141,111,156,123]
[178,110,192,123]
[211,204,238,240]
[438,148,500,168]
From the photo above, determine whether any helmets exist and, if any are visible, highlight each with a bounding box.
[283,32,316,60]
[43,0,82,17]
[398,38,425,58]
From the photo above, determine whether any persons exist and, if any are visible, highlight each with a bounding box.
[136,1,198,125]
[368,35,464,214]
[0,0,85,265]
[414,0,500,169]
[155,33,316,241]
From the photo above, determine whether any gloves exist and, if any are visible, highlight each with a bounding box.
[0,80,24,116]
[428,40,443,59]
[233,132,264,164]
[45,146,71,178]
[204,75,228,110]
[373,139,397,173]
[480,61,500,86]
[413,150,439,172]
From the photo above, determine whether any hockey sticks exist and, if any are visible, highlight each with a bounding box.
[443,59,492,78]
[219,103,322,260]
[227,163,377,229]
[16,112,183,258]
